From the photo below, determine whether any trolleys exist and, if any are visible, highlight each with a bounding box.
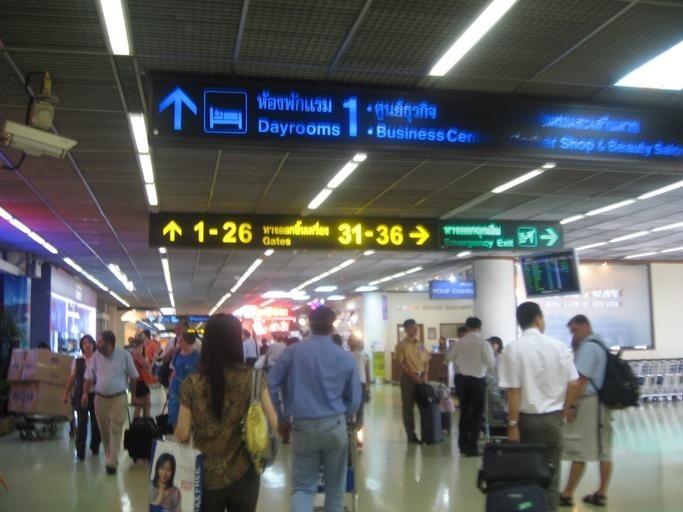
[283,415,356,512]
[626,355,683,406]
[10,386,77,442]
[483,380,512,448]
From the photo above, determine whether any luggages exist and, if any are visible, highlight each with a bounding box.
[123,395,156,463]
[422,408,443,444]
[485,485,547,510]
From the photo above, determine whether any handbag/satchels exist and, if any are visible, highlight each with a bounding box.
[157,358,173,386]
[239,367,279,476]
[476,439,556,487]
[157,398,176,435]
[414,381,444,406]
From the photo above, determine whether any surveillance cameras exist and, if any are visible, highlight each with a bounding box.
[0,120,78,160]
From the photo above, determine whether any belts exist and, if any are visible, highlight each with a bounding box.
[97,390,126,400]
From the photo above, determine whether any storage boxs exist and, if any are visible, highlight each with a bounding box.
[5,348,76,418]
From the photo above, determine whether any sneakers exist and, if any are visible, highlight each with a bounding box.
[405,432,421,445]
[77,443,119,476]
[281,433,291,444]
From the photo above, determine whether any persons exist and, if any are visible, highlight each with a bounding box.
[498,302,580,511]
[394,319,433,444]
[437,336,448,353]
[37,342,50,351]
[445,317,496,457]
[457,326,467,338]
[557,314,616,506]
[487,336,504,385]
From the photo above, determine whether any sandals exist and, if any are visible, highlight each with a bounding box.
[583,492,607,508]
[558,492,574,507]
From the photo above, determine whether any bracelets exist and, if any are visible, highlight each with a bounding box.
[570,405,577,412]
[507,420,519,427]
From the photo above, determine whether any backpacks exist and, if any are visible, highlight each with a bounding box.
[579,340,639,410]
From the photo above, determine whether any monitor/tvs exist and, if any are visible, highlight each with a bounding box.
[520,248,581,298]
